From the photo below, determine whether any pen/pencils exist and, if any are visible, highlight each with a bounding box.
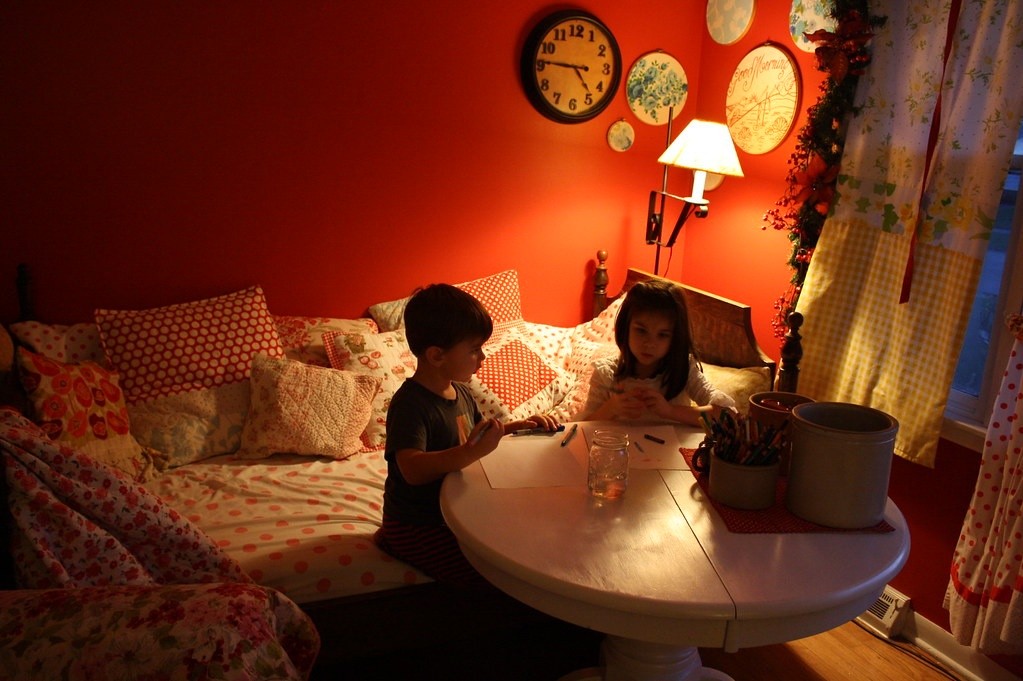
[699,409,792,465]
[469,411,503,447]
[513,426,565,436]
[561,424,577,446]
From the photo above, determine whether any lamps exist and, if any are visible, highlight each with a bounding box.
[646,106,745,278]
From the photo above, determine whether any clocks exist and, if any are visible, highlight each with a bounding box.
[520,9,622,124]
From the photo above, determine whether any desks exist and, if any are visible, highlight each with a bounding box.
[437,421,911,681]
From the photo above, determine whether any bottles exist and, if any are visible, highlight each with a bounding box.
[587,429,629,498]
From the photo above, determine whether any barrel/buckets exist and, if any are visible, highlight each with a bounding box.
[788,401,899,529]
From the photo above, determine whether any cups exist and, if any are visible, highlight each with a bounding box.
[709,446,781,509]
[692,436,715,480]
[749,391,817,475]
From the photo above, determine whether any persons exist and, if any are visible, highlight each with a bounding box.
[373,284,563,584]
[581,278,740,430]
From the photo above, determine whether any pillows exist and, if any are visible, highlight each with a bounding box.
[270,314,379,370]
[368,269,522,334]
[321,329,417,451]
[17,348,171,481]
[93,286,287,469]
[233,352,383,461]
[11,321,108,364]
[566,333,603,378]
[557,291,627,370]
[452,325,576,426]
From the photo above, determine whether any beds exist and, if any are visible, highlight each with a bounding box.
[0,250,805,681]
